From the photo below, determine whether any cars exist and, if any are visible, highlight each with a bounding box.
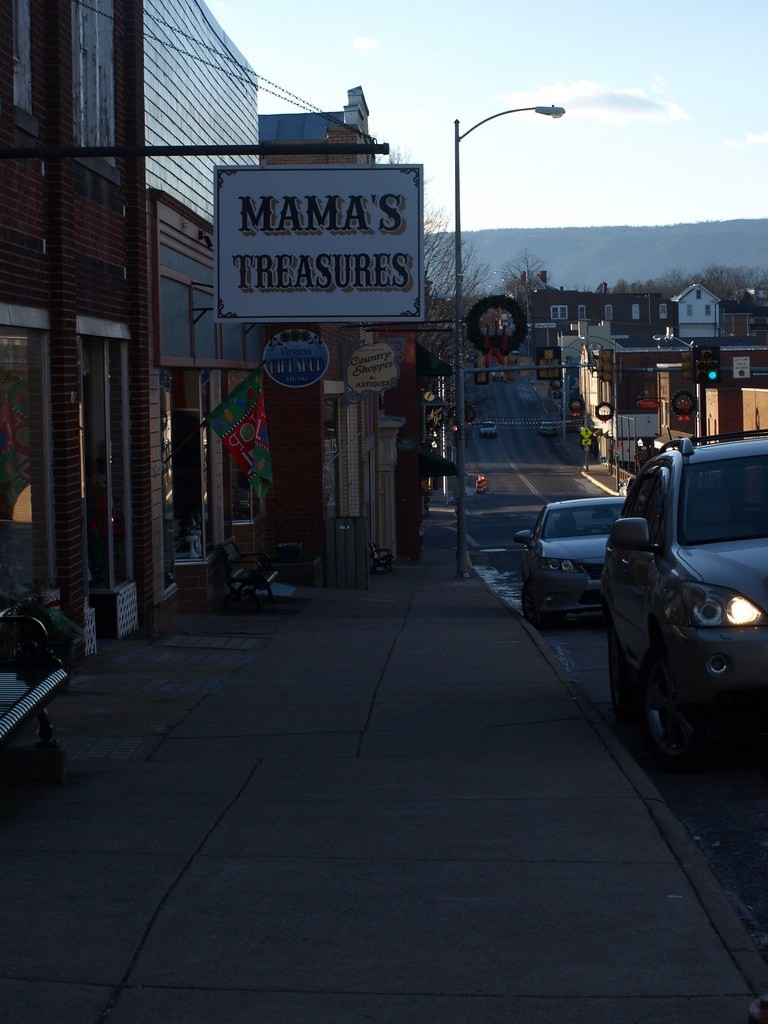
[512,496,626,626]
[600,439,768,744]
[479,421,497,438]
[539,422,555,435]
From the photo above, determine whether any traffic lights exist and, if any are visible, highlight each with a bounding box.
[598,348,624,385]
[692,346,721,388]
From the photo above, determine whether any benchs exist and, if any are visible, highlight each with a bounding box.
[369,543,393,575]
[216,535,279,610]
[0,608,70,785]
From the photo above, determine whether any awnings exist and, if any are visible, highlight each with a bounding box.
[416,343,454,376]
[424,397,446,406]
[419,449,457,479]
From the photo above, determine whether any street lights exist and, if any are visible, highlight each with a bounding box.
[453,105,566,577]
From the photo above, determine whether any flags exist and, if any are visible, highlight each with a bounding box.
[0,367,31,504]
[205,366,274,500]
